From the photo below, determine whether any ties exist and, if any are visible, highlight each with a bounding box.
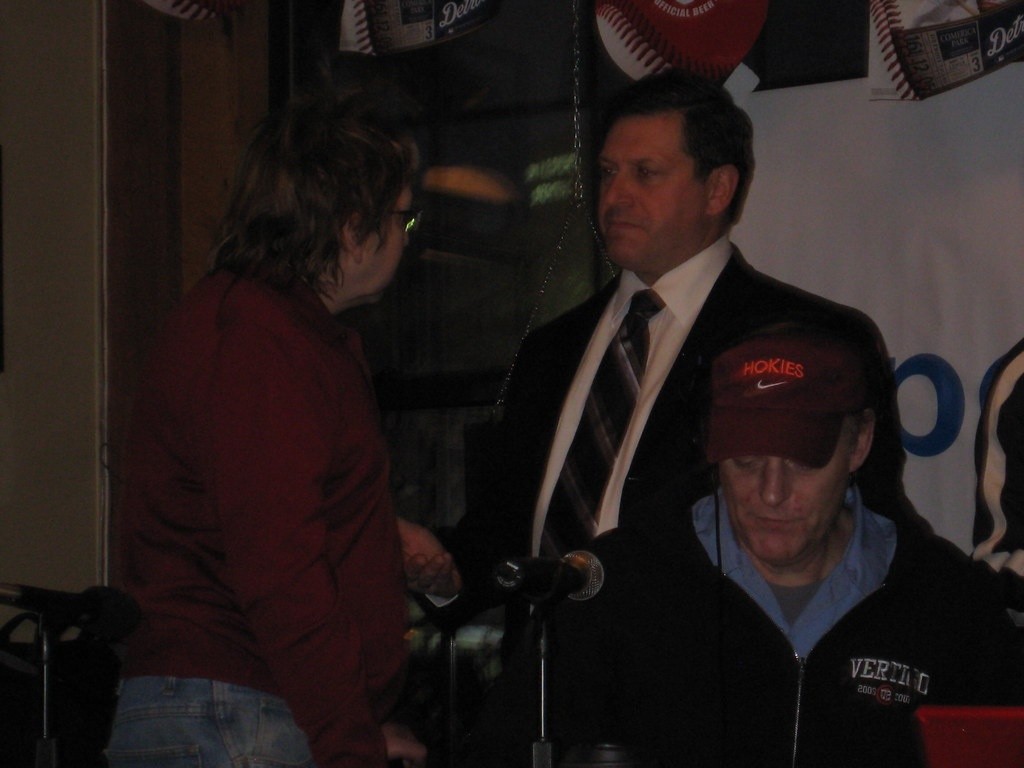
[538,289,666,569]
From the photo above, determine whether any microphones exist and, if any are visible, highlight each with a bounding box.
[495,552,605,601]
[0,580,141,640]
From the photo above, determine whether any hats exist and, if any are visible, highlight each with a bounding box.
[705,331,870,472]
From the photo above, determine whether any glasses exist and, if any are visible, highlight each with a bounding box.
[390,206,424,235]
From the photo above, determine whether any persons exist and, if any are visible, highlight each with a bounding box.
[109,98,430,768]
[398,71,906,768]
[582,338,1024,767]
